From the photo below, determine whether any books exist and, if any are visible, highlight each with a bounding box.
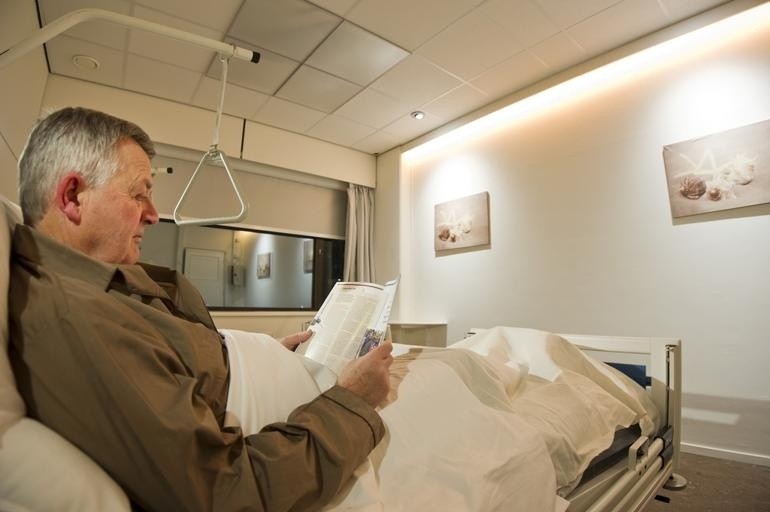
[295,275,400,374]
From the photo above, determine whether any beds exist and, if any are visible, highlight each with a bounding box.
[0,196,686,510]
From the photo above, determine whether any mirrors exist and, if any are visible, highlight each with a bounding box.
[134,217,316,311]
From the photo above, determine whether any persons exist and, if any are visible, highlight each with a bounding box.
[8,105,395,512]
